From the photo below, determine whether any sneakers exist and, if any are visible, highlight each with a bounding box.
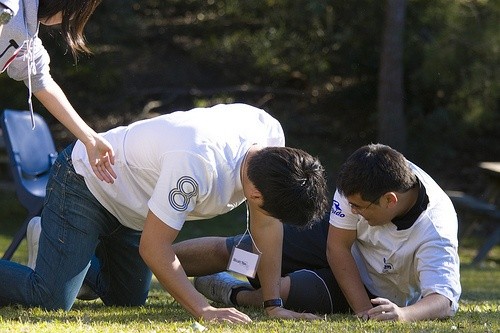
[193,271,255,306]
[26,215,42,272]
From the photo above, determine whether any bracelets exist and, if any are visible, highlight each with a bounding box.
[362,313,370,322]
[262,298,283,310]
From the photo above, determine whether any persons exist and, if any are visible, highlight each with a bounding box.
[0,0,118,184]
[77,142,461,321]
[0,103,328,324]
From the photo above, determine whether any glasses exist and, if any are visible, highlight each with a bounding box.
[351,192,386,216]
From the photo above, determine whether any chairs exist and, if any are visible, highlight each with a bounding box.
[1,110,59,260]
[446,186,500,266]
